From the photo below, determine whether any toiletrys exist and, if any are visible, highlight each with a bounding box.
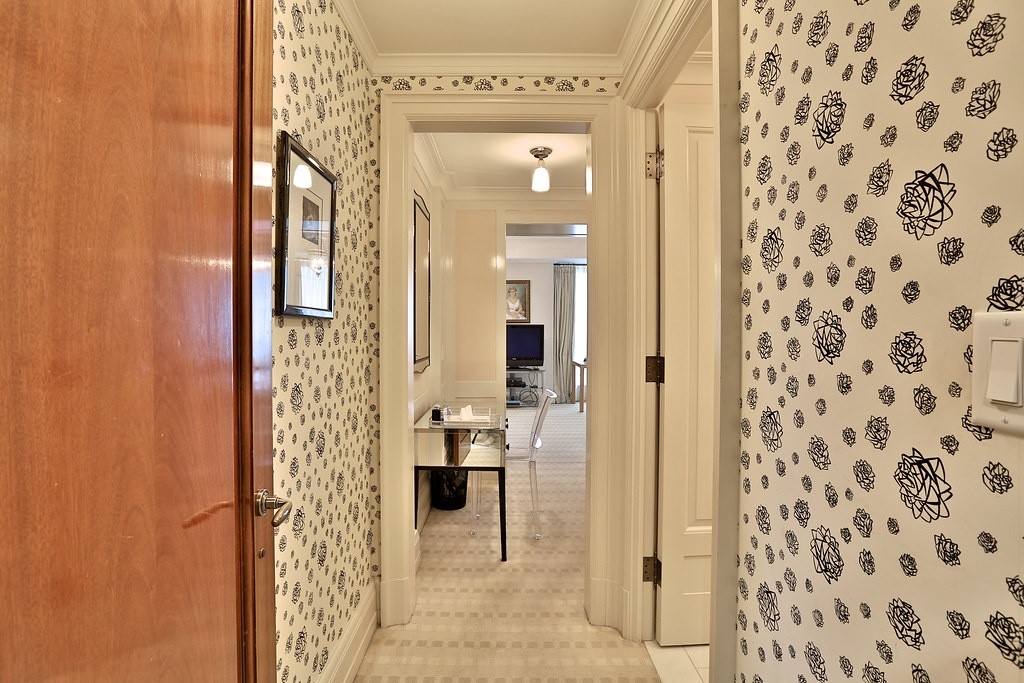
[431,405,452,426]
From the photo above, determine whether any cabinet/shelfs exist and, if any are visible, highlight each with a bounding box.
[505,368,545,407]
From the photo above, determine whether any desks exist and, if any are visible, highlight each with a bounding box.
[571,359,588,412]
[413,401,508,561]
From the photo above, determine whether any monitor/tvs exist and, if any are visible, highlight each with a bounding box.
[506,324,544,371]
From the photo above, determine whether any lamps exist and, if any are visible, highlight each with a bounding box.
[529,146,553,192]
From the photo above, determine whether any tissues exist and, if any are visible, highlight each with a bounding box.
[443,405,488,426]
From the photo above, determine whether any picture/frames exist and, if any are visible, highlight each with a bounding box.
[273,129,337,320]
[413,189,430,374]
[505,280,530,323]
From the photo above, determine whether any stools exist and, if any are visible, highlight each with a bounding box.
[470,387,559,540]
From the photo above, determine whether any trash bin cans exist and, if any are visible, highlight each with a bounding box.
[430,470,468,512]
